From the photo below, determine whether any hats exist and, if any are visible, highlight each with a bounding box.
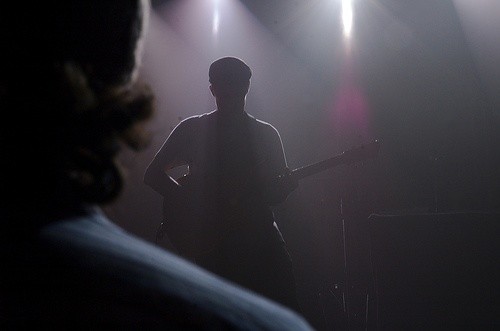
[209,56,252,83]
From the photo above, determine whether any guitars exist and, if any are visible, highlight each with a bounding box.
[161,138,379,252]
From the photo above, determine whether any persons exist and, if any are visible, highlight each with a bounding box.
[142,55,302,311]
[0,0,315,330]
